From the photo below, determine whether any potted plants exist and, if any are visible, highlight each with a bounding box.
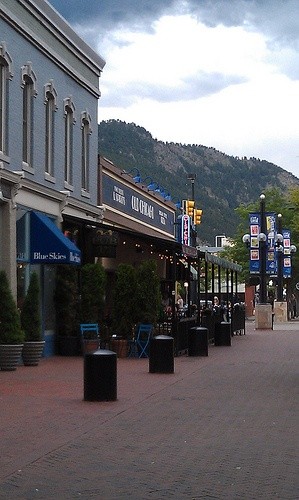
[55,258,162,359]
[20,270,46,366]
[0,270,24,372]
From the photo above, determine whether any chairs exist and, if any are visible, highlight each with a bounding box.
[80,323,104,351]
[127,323,152,359]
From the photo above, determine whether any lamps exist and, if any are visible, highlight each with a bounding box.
[120,167,181,208]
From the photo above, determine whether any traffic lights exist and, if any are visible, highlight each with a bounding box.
[186,200,195,216]
[194,209,202,225]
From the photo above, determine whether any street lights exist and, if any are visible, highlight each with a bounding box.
[242,194,297,306]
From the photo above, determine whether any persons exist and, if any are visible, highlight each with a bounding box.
[255,289,275,310]
[200,296,231,326]
[176,295,183,308]
[233,293,240,303]
[184,301,197,318]
[288,288,299,321]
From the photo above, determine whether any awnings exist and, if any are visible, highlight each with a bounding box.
[29,211,81,266]
[60,213,198,259]
[197,250,242,273]
[16,210,29,263]
